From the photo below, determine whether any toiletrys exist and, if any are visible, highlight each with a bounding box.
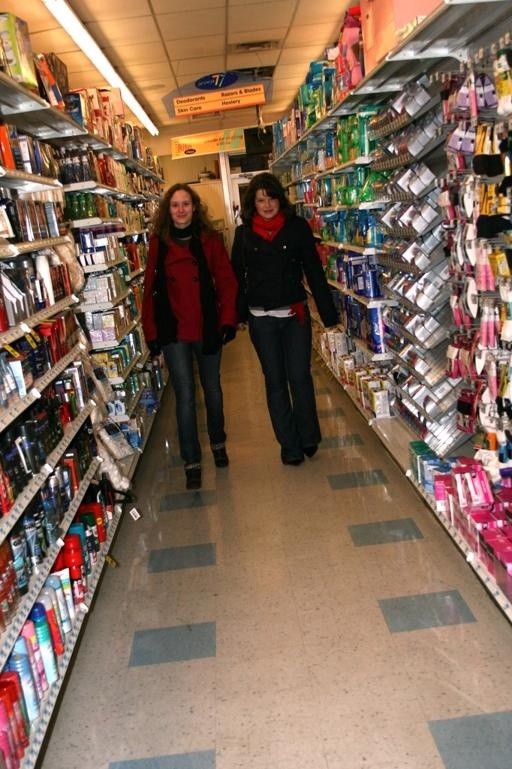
[0,361,105,769]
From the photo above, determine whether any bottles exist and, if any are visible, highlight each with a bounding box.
[51,144,142,195]
[65,193,134,219]
[0,392,63,511]
[0,455,74,769]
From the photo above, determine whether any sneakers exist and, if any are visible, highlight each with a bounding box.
[210,443,230,468]
[285,444,319,466]
[184,462,202,491]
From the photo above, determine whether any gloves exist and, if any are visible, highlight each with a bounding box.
[220,325,237,345]
[146,340,161,356]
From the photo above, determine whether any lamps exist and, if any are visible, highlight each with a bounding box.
[42,0,159,137]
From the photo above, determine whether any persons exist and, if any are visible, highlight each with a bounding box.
[140,183,237,490]
[229,172,341,467]
